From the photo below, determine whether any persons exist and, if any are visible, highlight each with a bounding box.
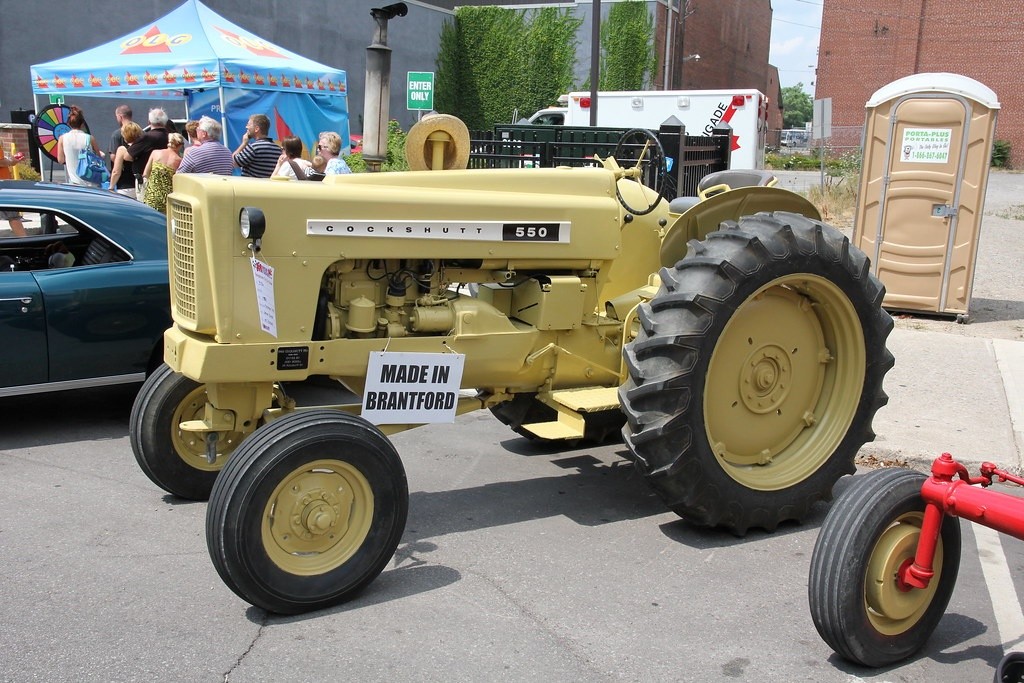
[304,155,327,180]
[122,107,169,206]
[269,136,312,180]
[232,114,283,178]
[141,132,184,216]
[183,121,202,157]
[56,107,102,188]
[316,130,354,175]
[175,114,234,176]
[107,122,143,200]
[108,104,132,173]
[0,139,27,236]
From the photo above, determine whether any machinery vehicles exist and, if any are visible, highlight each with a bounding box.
[129,126,897,618]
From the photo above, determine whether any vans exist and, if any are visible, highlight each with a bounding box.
[781,128,809,148]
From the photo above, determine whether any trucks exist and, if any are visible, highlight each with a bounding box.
[471,89,769,175]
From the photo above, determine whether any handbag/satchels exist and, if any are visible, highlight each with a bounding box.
[75,134,110,183]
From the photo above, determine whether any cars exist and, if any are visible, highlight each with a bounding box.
[0,178,169,412]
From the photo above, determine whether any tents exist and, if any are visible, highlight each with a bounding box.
[28,0,350,177]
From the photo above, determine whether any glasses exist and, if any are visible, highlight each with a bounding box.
[318,145,328,150]
